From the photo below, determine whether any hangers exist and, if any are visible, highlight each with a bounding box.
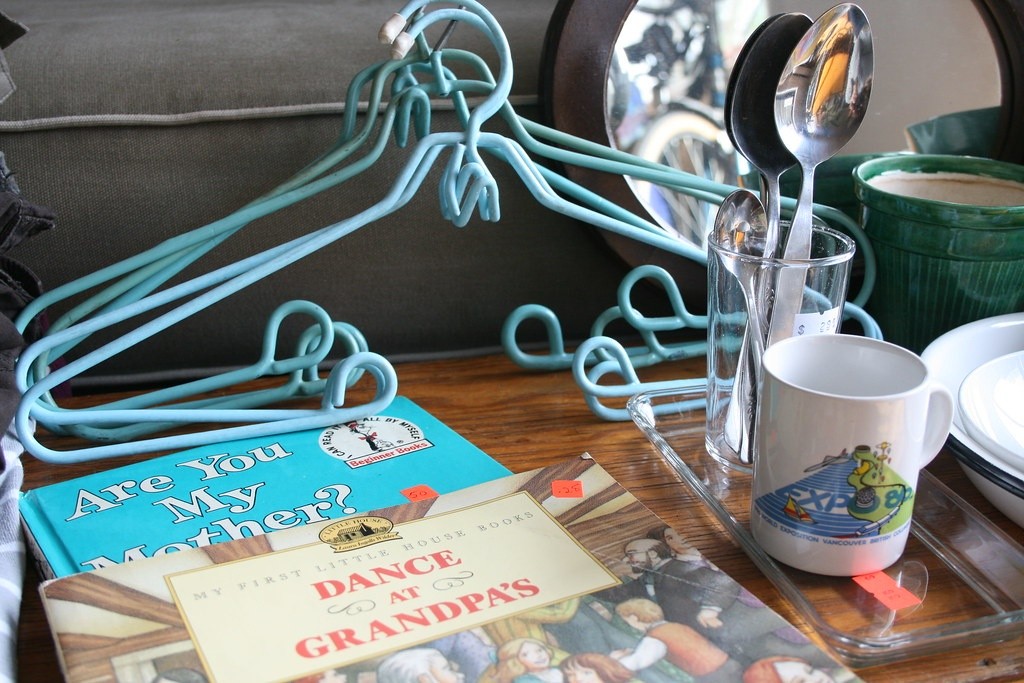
[16,0,882,463]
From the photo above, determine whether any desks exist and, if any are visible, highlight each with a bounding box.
[10,349,1024,683]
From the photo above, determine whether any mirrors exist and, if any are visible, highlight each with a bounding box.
[538,0,1024,313]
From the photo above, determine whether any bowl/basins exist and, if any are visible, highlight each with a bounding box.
[920,313,1023,531]
[957,351,1023,474]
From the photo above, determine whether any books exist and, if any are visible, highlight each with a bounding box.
[15,393,869,683]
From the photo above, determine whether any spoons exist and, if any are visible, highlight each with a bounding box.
[715,3,874,262]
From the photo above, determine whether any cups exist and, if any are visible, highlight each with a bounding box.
[751,333,954,577]
[707,222,856,473]
[853,154,1024,354]
